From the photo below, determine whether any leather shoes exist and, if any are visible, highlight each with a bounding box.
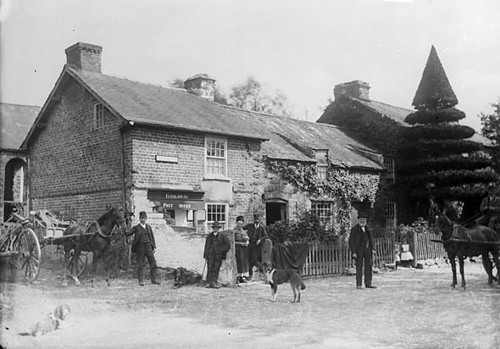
[211,282,219,288]
[139,282,144,286]
[151,281,160,284]
[366,285,376,288]
[357,286,364,289]
[205,283,210,288]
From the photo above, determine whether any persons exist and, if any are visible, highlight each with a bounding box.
[124,211,161,286]
[203,220,231,289]
[242,219,269,282]
[231,215,250,284]
[478,181,500,229]
[348,211,378,289]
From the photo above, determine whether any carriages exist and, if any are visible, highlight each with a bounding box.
[0,206,130,288]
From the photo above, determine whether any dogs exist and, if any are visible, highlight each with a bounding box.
[172,266,203,289]
[261,260,306,303]
[18,303,72,338]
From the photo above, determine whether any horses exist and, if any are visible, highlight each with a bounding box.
[60,205,130,288]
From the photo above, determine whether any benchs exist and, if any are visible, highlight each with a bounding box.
[31,212,69,237]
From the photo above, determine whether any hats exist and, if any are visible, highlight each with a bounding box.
[357,211,368,219]
[211,221,222,228]
[254,214,259,219]
[236,216,244,221]
[140,212,147,219]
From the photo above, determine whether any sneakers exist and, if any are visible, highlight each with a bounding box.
[239,277,247,283]
[248,276,252,281]
